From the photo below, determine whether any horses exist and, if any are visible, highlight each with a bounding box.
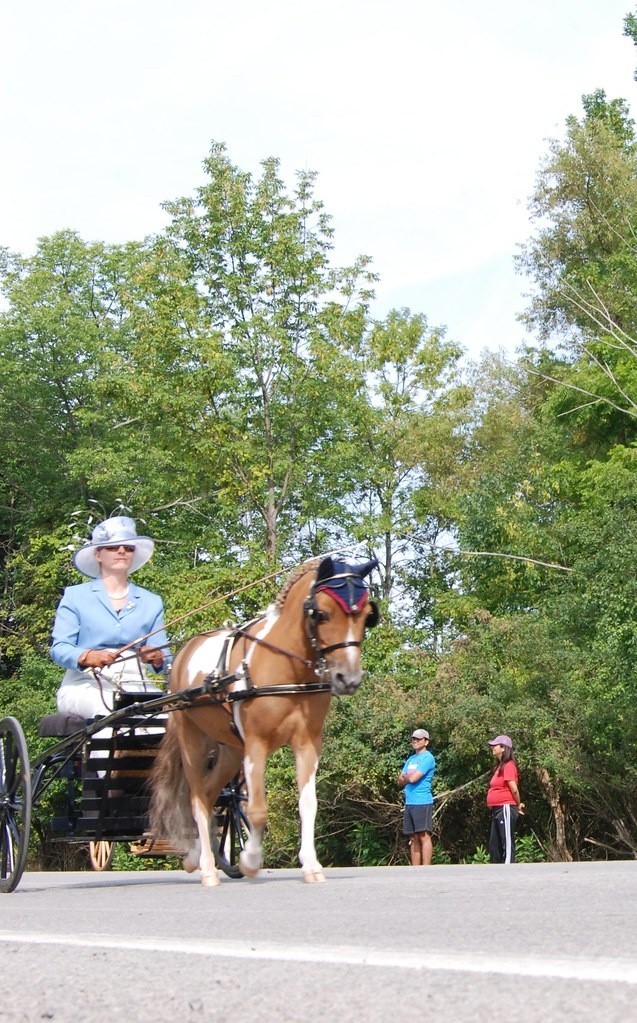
[146,555,380,887]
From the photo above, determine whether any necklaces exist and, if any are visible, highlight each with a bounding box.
[105,580,129,599]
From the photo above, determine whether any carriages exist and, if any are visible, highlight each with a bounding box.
[1,554,390,894]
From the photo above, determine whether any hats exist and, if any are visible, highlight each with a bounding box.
[73,516,155,579]
[488,735,512,748]
[411,729,429,740]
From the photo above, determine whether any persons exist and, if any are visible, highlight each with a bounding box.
[395,729,436,866]
[485,734,525,864]
[48,516,175,797]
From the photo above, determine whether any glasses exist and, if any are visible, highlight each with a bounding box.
[97,544,135,552]
